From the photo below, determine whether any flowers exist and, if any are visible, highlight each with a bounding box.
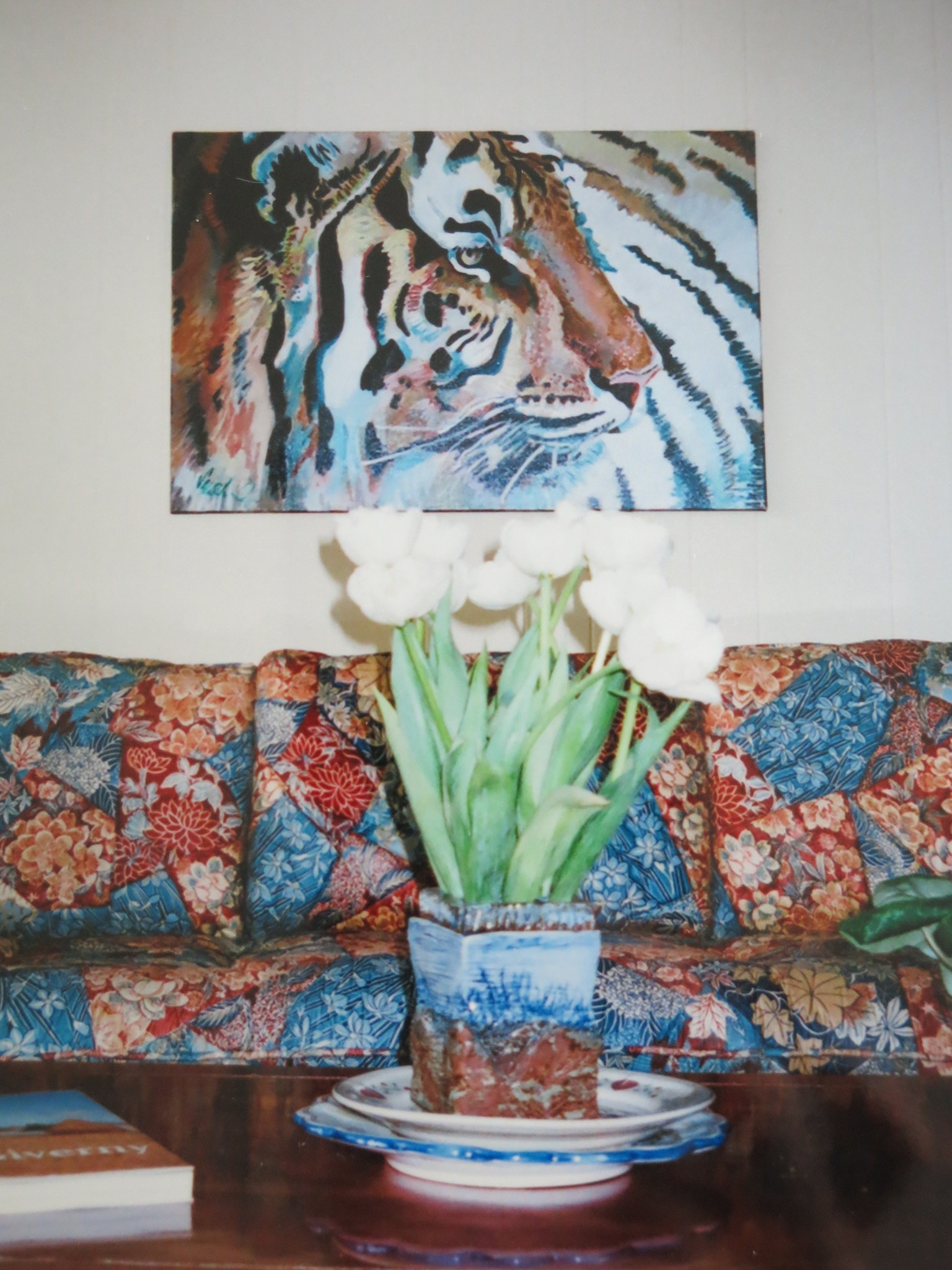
[340,490,728,907]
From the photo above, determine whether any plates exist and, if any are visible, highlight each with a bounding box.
[331,1064,716,1153]
[290,1091,727,1190]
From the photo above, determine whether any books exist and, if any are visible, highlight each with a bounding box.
[0,1088,195,1213]
[0,1203,197,1250]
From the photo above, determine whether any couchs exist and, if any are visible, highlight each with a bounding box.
[0,640,952,1075]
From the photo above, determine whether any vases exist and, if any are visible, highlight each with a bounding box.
[407,887,603,1119]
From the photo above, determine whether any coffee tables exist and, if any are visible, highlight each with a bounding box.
[0,1061,952,1270]
